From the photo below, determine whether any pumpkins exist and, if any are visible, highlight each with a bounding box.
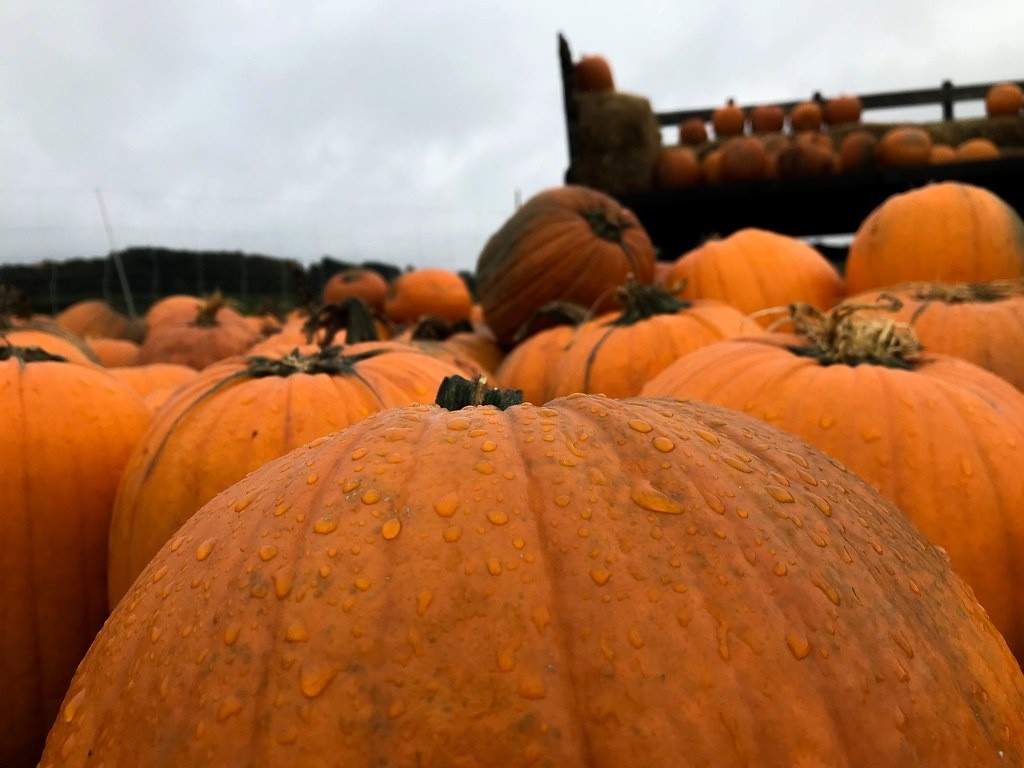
[0,49,1024,768]
